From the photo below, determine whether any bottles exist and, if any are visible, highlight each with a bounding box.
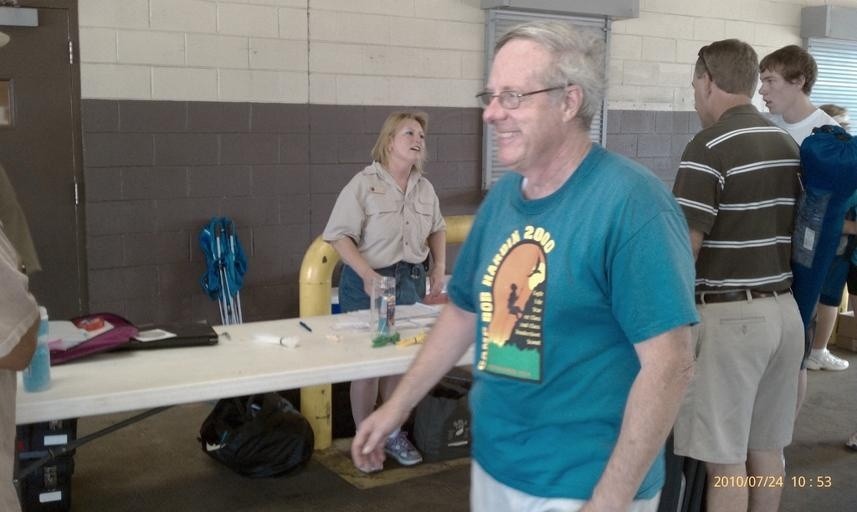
[21,307,52,392]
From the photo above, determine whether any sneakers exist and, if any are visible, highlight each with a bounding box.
[803,348,849,372]
[383,432,424,468]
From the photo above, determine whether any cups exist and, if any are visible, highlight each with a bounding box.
[369,277,397,341]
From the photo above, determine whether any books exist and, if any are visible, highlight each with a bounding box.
[358,297,440,331]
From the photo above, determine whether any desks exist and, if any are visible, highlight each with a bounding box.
[14,300,476,488]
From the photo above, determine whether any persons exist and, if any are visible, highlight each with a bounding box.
[667,37,804,512]
[349,22,701,512]
[0,223,40,511]
[756,45,841,170]
[806,100,856,376]
[320,107,448,467]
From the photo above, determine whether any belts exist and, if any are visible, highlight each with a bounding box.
[694,286,794,305]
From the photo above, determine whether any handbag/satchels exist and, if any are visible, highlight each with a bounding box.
[196,389,316,482]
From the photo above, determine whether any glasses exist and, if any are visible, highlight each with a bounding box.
[474,83,567,112]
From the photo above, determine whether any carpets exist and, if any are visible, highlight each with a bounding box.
[312,427,470,491]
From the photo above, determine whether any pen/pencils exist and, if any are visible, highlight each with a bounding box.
[300,322,312,331]
